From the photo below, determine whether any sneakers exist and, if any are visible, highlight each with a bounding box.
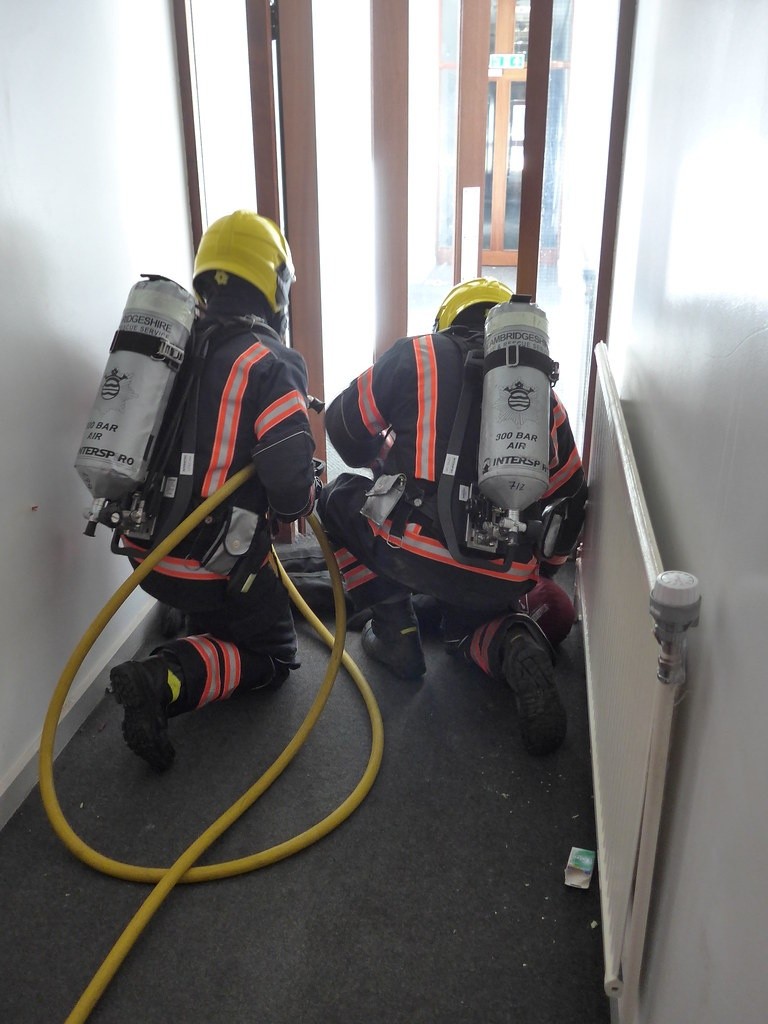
[158,597,190,637]
[498,623,568,758]
[362,618,426,680]
[109,653,186,768]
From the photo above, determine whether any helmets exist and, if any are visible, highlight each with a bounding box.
[433,275,516,334]
[192,211,296,314]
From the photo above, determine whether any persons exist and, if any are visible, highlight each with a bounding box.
[317,278,588,756]
[110,211,323,773]
